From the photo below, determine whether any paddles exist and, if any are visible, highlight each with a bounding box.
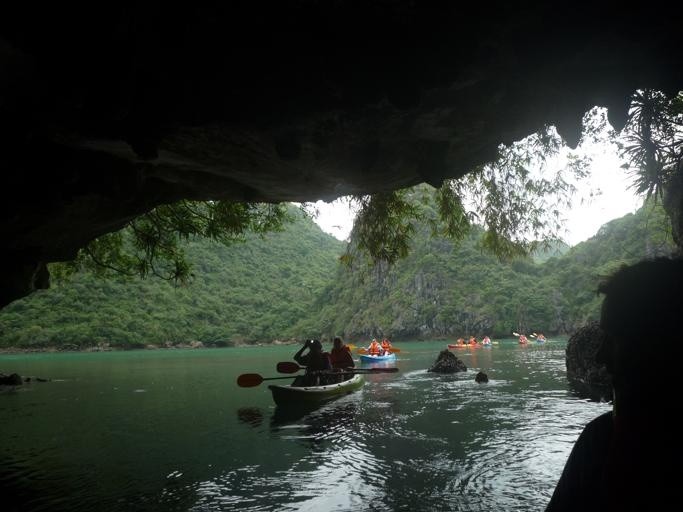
[237,370,399,387]
[513,333,521,337]
[277,362,399,373]
[346,344,369,349]
[357,349,400,353]
[529,333,538,338]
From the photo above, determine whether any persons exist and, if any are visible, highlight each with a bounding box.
[380,339,392,356]
[293,338,333,387]
[456,335,490,345]
[367,338,384,356]
[328,336,355,380]
[542,256,683,512]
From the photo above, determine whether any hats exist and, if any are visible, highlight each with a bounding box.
[306,340,323,352]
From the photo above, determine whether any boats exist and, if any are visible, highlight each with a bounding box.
[450,341,498,348]
[268,374,365,407]
[360,351,395,365]
[519,335,546,345]
